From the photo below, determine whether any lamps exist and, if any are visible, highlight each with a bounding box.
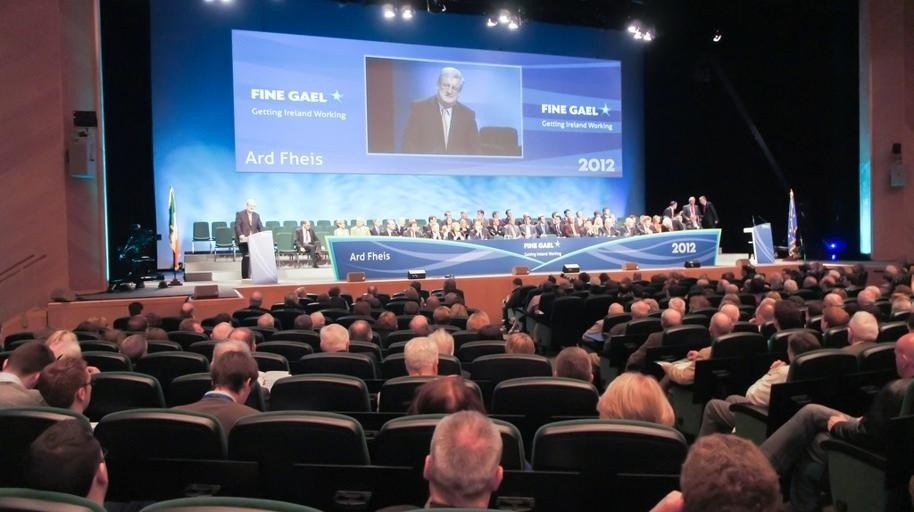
[486,2,524,31]
[382,0,414,20]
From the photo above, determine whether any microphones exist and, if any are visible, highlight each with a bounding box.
[256,223,262,232]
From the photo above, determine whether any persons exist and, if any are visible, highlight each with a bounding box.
[644,432,785,512]
[596,371,675,428]
[399,66,481,154]
[294,220,324,267]
[26,417,112,511]
[0,339,55,409]
[367,408,507,511]
[333,207,700,241]
[841,309,878,354]
[693,331,821,438]
[412,374,489,418]
[168,347,261,433]
[37,355,92,412]
[233,198,264,279]
[664,195,718,229]
[755,330,914,510]
[47,263,914,408]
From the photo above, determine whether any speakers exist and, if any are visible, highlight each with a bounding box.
[623,262,640,270]
[512,266,530,275]
[347,271,367,281]
[891,141,903,163]
[195,285,219,298]
[72,110,98,129]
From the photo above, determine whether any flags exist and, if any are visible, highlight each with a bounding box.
[786,191,801,257]
[167,188,184,273]
